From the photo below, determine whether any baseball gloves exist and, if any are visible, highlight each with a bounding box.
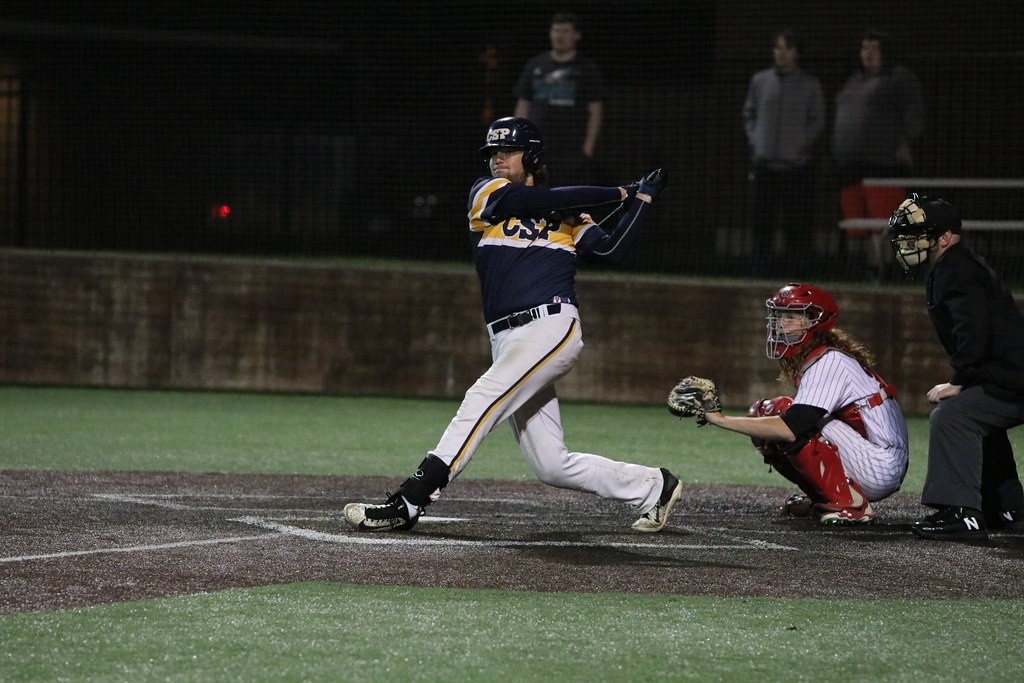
[666,374,723,429]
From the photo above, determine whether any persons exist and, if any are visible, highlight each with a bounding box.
[831,26,924,191]
[887,191,1024,539]
[511,12,610,156]
[738,25,826,280]
[668,282,909,525]
[343,117,682,533]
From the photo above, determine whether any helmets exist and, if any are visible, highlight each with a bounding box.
[766,282,839,359]
[480,116,544,172]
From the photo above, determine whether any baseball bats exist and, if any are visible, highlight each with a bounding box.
[598,168,667,229]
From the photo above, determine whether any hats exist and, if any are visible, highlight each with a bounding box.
[924,198,962,235]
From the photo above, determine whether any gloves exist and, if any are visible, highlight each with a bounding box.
[623,168,672,203]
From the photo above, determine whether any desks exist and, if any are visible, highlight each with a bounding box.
[862,177,1024,281]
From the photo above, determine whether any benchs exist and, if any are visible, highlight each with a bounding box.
[839,218,1024,280]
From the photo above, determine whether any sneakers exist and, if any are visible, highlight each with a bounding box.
[784,494,826,516]
[821,498,876,526]
[343,492,426,533]
[982,506,1024,534]
[631,467,682,532]
[912,506,988,545]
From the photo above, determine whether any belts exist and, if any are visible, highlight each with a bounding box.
[906,460,909,471]
[492,303,562,335]
